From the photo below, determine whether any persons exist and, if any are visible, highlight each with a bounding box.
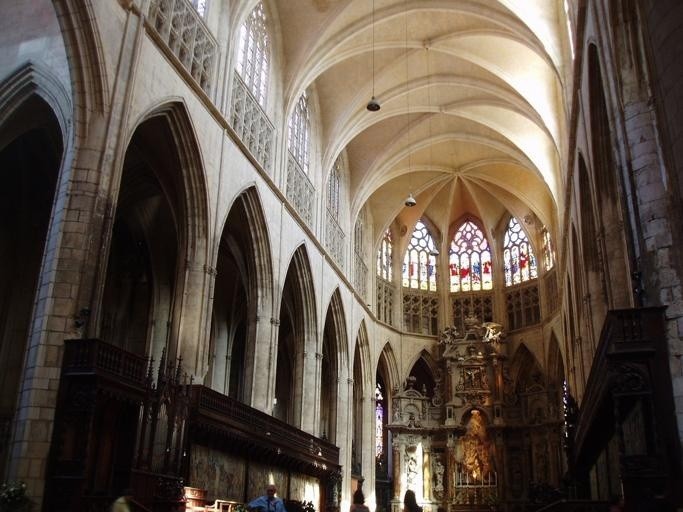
[402,490,423,512]
[246,484,286,512]
[111,487,137,512]
[349,490,369,512]
[435,461,445,485]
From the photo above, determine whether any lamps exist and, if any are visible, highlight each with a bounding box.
[366,1,382,112]
[405,0,416,207]
